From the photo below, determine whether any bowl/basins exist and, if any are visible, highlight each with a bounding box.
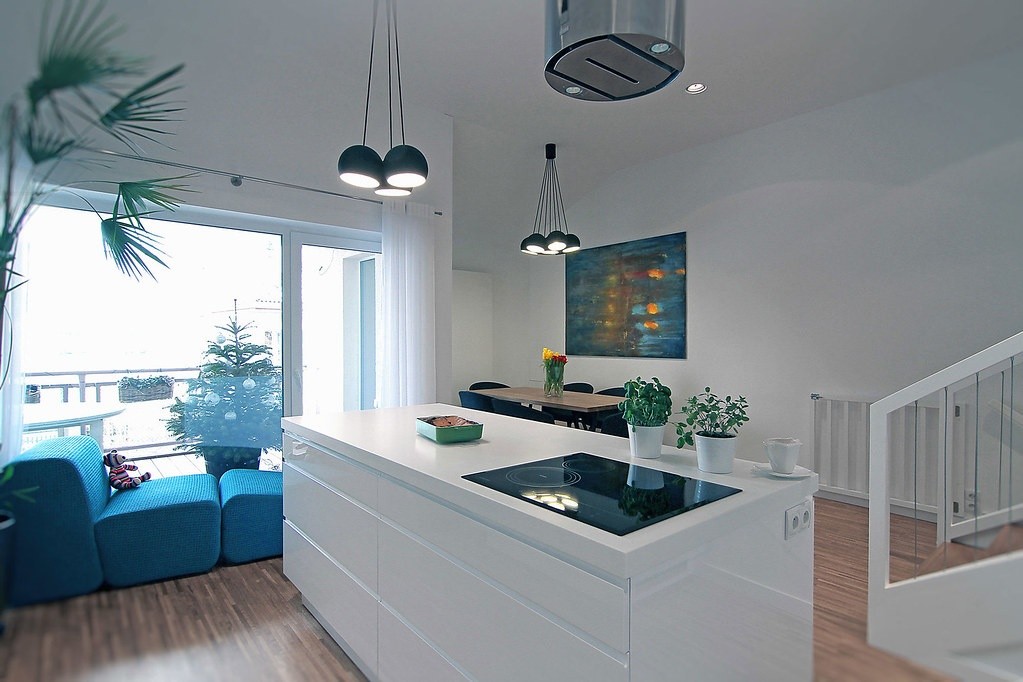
[416,416,483,442]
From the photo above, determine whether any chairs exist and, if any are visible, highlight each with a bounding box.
[459,382,630,440]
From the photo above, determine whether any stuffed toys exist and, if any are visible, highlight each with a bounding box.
[102,449,151,490]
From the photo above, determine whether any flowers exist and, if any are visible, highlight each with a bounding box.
[541,347,568,396]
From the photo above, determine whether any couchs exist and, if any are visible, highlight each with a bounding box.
[0,436,283,606]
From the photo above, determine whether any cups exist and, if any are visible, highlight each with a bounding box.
[763,437,802,473]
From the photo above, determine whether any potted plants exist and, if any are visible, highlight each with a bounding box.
[616,376,672,459]
[162,298,286,479]
[674,387,749,473]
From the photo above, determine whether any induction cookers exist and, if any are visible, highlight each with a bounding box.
[462,450,742,537]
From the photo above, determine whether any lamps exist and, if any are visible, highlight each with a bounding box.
[520,144,582,254]
[337,0,428,197]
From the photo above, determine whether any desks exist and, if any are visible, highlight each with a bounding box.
[280,403,819,682]
[23,401,124,455]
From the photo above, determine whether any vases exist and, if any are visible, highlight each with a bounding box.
[543,365,564,398]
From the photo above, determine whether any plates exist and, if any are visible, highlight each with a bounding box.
[754,462,813,477]
